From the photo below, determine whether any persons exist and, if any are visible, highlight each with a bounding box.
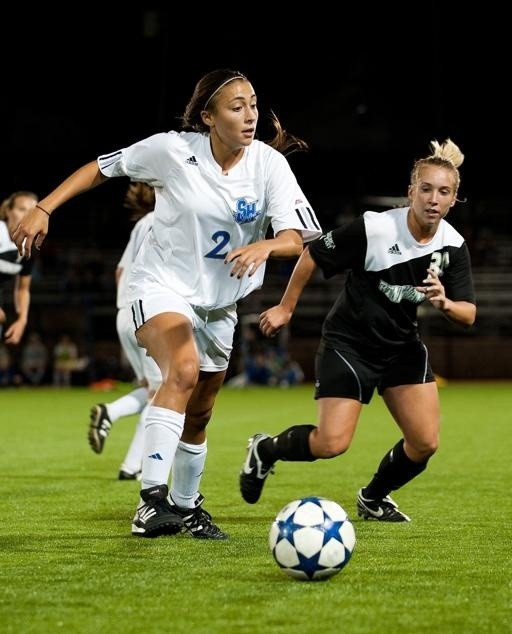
[19,330,49,386]
[1,188,40,347]
[7,69,325,540]
[87,182,160,479]
[238,135,479,524]
[51,334,79,386]
[221,311,306,390]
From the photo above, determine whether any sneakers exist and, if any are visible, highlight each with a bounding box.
[357,487,412,522]
[166,490,230,540]
[119,463,143,481]
[239,432,274,504]
[132,484,184,538]
[88,403,112,454]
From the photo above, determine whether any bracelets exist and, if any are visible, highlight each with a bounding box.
[34,204,50,216]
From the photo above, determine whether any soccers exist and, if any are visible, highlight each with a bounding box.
[269,496,356,581]
